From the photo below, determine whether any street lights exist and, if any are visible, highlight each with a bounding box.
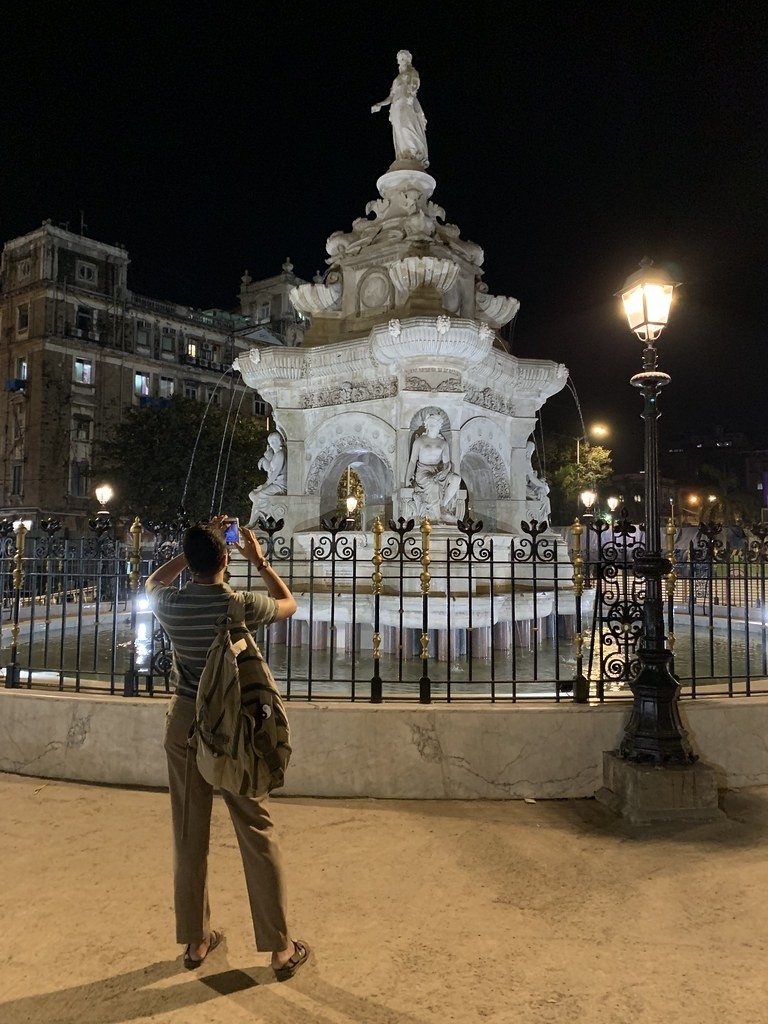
[572,424,608,525]
[716,441,732,527]
[92,482,115,603]
[690,496,701,526]
[614,256,701,769]
[579,489,596,590]
[606,496,620,573]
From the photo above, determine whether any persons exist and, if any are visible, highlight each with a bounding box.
[372,50,430,169]
[526,440,556,533]
[144,512,310,983]
[243,432,287,529]
[405,414,462,525]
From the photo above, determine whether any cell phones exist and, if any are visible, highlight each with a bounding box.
[221,516,239,549]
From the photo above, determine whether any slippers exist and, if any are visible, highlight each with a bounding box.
[184,929,223,970]
[274,939,311,982]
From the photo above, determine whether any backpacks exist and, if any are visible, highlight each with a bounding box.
[186,592,293,798]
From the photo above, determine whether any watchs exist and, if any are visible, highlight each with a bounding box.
[257,559,271,571]
